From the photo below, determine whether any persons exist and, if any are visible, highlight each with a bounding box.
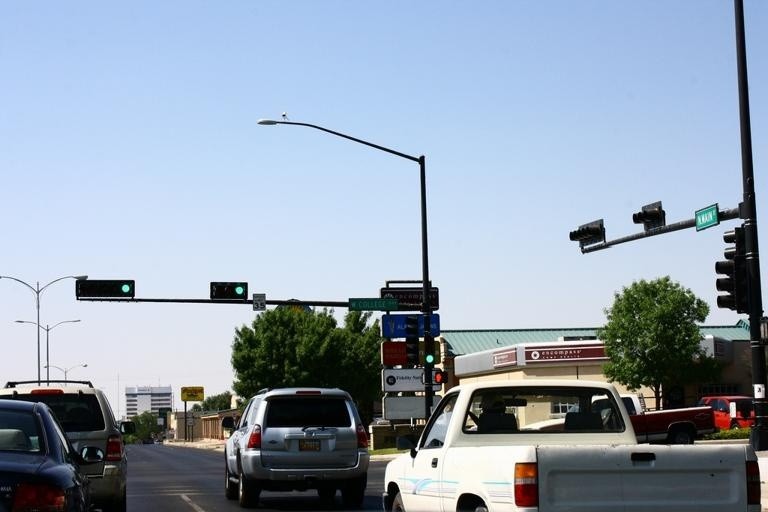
[468,392,507,434]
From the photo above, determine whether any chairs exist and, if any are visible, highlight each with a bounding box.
[481,413,517,434]
[566,409,604,429]
[0,430,34,452]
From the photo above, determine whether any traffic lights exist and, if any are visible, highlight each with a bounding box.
[713,260,748,311]
[258,119,434,422]
[723,226,747,260]
[75,280,135,297]
[425,338,436,369]
[569,220,605,245]
[435,371,448,383]
[210,281,248,300]
[631,199,665,231]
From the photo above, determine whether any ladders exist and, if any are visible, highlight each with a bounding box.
[638,393,646,412]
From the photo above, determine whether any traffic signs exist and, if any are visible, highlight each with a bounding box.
[693,201,720,232]
[348,297,397,311]
[252,292,267,311]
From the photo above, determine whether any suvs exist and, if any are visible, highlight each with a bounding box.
[222,387,370,508]
[0,380,137,512]
[697,395,755,431]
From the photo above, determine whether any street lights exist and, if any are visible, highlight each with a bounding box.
[0,273,88,386]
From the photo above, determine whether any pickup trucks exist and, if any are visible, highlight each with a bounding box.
[382,383,760,512]
[521,393,719,445]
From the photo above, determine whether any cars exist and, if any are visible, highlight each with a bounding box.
[0,398,105,511]
